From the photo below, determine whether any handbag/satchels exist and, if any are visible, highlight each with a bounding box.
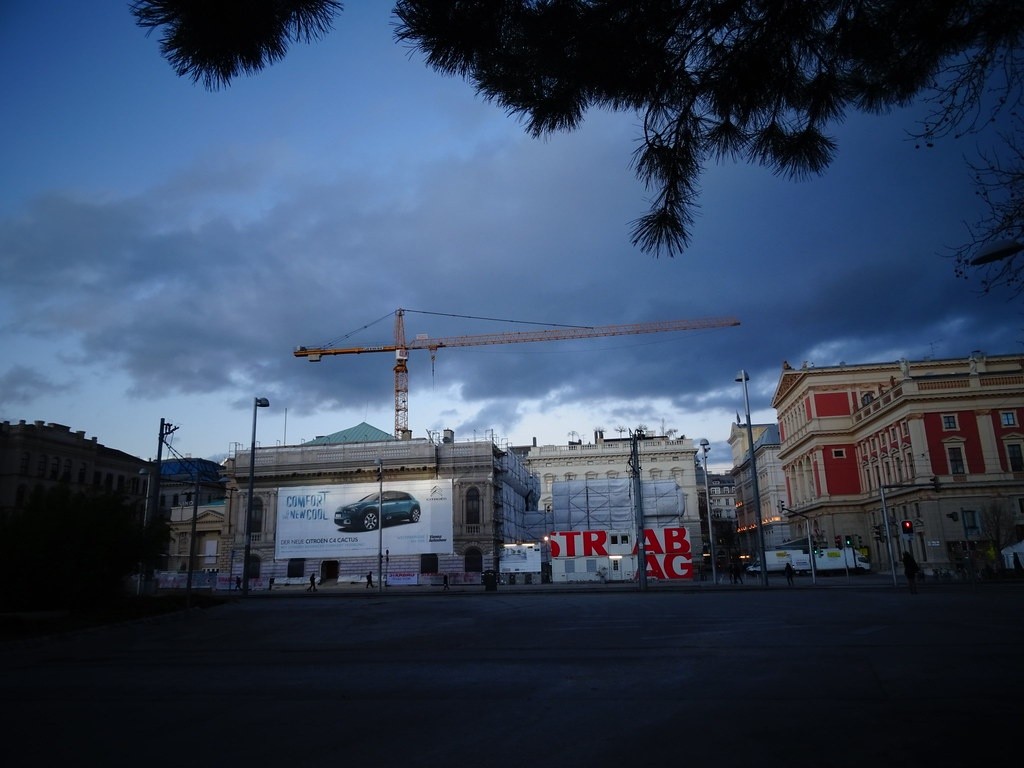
[908,556,920,573]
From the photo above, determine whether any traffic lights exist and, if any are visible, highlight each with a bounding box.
[845,535,853,547]
[902,520,913,534]
[814,546,818,555]
[834,535,843,550]
[817,547,824,558]
[854,535,863,549]
[777,500,786,512]
[930,476,940,493]
[874,525,884,542]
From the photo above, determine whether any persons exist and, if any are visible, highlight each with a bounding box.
[443,573,449,590]
[701,566,707,581]
[975,567,984,577]
[307,573,318,591]
[734,564,743,584]
[366,571,374,588]
[235,576,243,590]
[918,567,969,584]
[785,563,793,586]
[903,551,919,594]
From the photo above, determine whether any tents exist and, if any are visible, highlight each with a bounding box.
[1000,540,1024,569]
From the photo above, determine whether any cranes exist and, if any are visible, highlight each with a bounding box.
[291,306,743,440]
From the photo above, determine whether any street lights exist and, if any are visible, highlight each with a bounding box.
[136,467,152,598]
[698,438,720,586]
[240,397,270,598]
[373,457,384,592]
[734,369,770,590]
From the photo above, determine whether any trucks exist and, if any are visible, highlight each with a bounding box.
[814,547,871,573]
[745,549,813,575]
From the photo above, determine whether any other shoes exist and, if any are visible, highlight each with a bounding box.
[915,592,918,594]
[911,592,914,594]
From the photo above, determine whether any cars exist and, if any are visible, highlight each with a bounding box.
[333,489,421,530]
